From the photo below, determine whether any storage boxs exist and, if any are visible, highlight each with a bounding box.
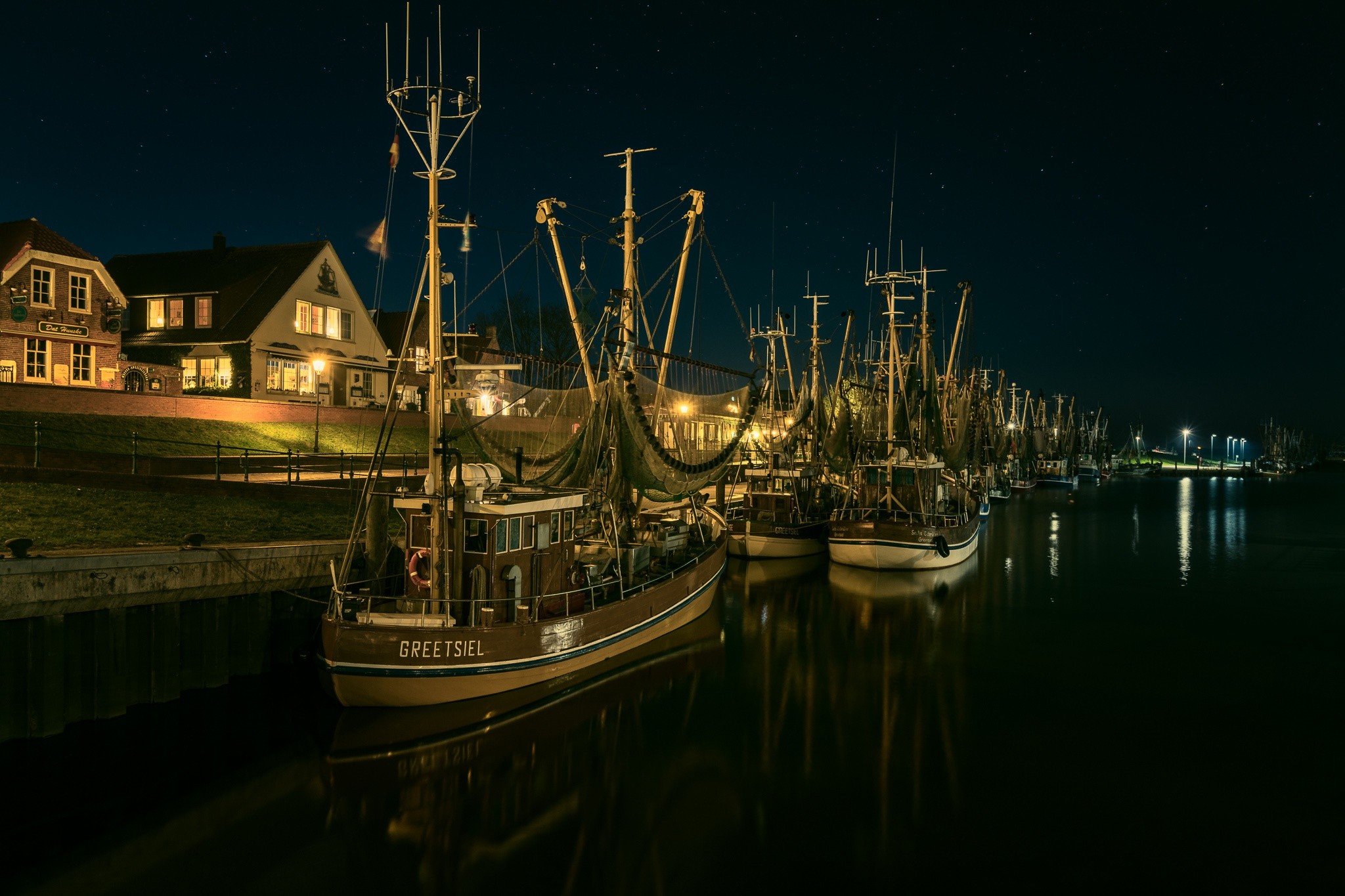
[396,600,430,615]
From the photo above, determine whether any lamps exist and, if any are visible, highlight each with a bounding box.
[106,296,114,309]
[10,282,17,296]
[20,282,28,294]
[114,297,121,307]
[47,310,54,321]
[79,314,85,324]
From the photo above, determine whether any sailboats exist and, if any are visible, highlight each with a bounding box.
[1242,415,1320,478]
[727,143,1163,569]
[810,553,983,842]
[316,0,761,710]
[318,594,746,895]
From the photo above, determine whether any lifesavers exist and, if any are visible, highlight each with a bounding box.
[408,549,433,587]
[937,535,950,558]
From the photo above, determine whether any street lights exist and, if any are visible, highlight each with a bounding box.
[313,360,326,454]
[1232,438,1238,460]
[1240,438,1247,460]
[1211,434,1217,462]
[1183,429,1188,465]
[1227,436,1233,463]
